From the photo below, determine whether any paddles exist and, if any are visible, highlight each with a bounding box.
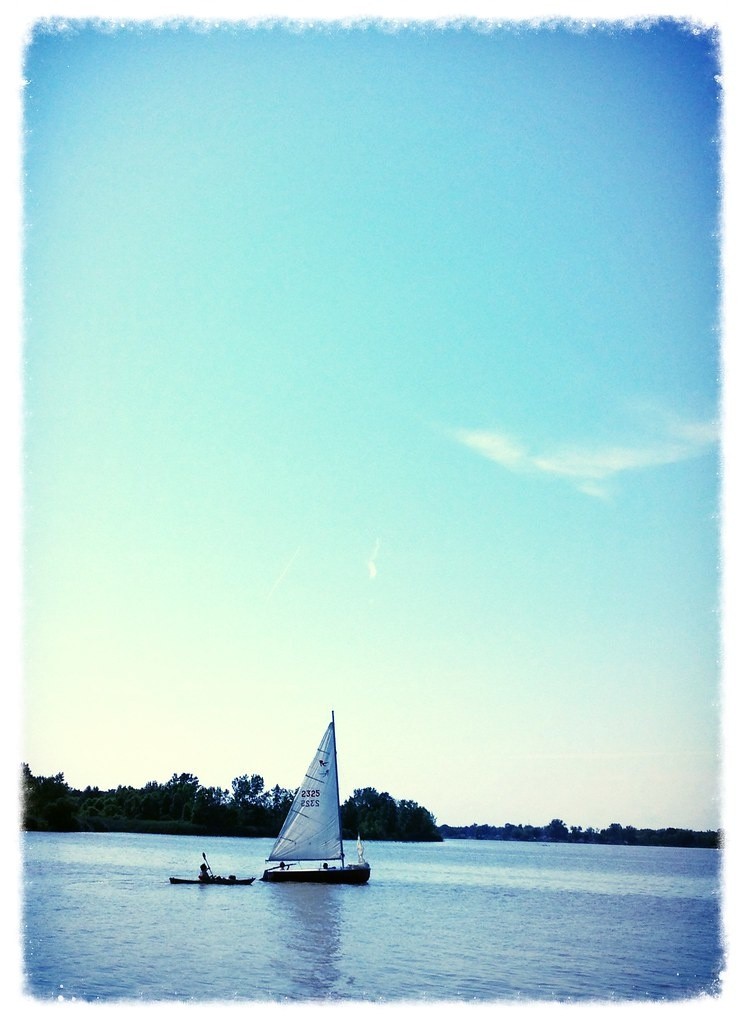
[202,851,214,877]
[266,862,297,871]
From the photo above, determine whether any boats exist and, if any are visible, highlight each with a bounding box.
[168,876,257,887]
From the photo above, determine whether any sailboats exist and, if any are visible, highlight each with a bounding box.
[263,710,371,887]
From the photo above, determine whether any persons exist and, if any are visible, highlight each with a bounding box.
[323,863,329,869]
[198,863,212,881]
[280,862,285,870]
